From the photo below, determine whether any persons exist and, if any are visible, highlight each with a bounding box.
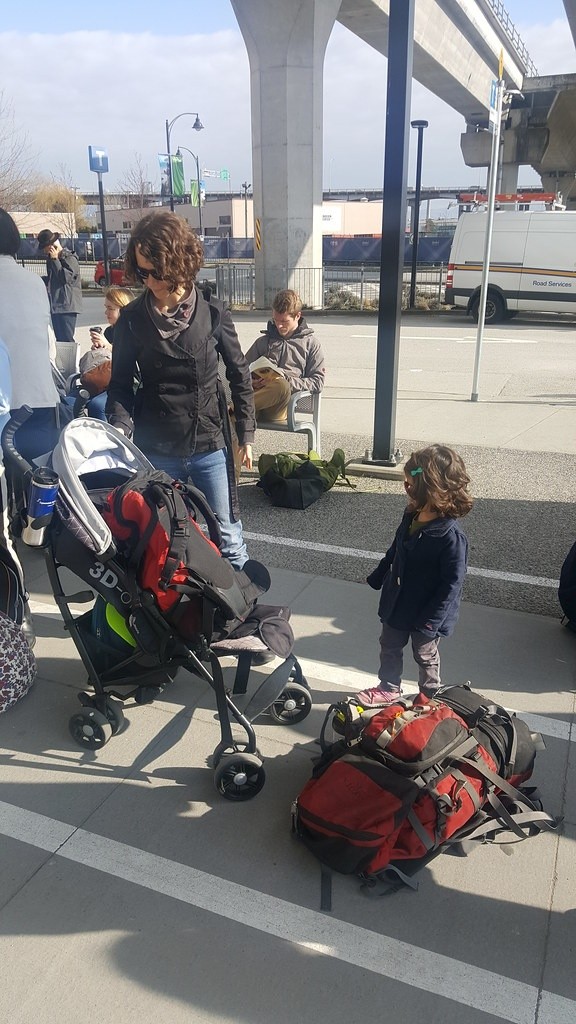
[89,284,138,354]
[228,289,327,485]
[103,210,256,569]
[0,204,61,535]
[353,445,474,709]
[31,229,80,344]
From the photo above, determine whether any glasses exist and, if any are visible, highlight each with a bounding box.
[134,265,169,280]
[404,480,417,500]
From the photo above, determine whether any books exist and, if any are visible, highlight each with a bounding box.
[245,355,284,391]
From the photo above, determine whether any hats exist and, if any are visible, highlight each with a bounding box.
[38,229,59,249]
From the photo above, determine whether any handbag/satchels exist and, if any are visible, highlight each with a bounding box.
[258,449,357,509]
[0,610,37,714]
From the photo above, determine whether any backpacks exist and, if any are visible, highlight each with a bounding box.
[289,680,563,909]
[0,546,29,625]
[99,467,271,650]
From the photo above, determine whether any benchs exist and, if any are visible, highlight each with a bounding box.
[66,353,321,456]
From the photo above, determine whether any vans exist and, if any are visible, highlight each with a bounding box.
[441,203,576,324]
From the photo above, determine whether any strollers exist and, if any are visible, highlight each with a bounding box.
[1,388,313,801]
[49,359,113,426]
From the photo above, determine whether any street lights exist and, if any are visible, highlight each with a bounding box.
[242,181,251,238]
[472,80,525,400]
[166,112,204,212]
[410,119,429,308]
[71,186,80,210]
[175,146,203,235]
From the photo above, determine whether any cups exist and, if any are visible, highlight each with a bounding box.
[89,327,102,343]
[20,467,59,546]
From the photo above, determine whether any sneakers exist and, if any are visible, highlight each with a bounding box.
[355,686,401,707]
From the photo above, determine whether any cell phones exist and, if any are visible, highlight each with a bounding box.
[50,246,57,252]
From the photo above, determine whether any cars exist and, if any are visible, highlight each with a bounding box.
[95,259,134,287]
